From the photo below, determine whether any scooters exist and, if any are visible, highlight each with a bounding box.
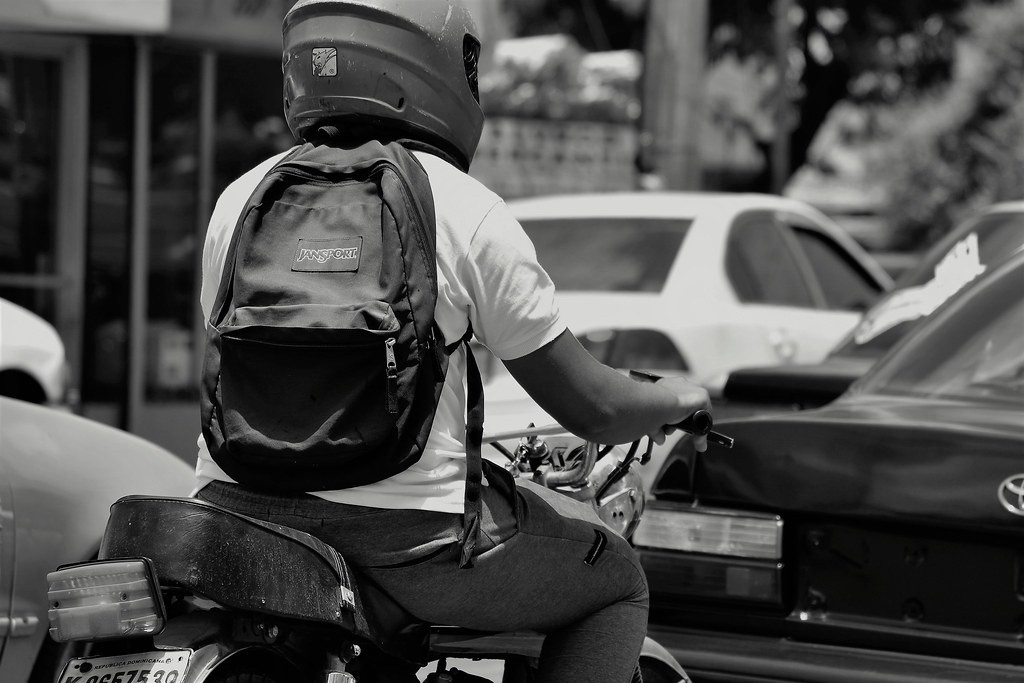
[35,397,736,681]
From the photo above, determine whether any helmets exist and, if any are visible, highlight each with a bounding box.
[282,0,487,175]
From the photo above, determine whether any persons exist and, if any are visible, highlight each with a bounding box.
[194,1,713,683]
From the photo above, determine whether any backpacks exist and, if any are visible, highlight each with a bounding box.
[199,136,450,495]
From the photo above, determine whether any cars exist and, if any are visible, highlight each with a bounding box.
[2,297,214,683]
[722,206,1023,420]
[485,198,902,469]
[631,256,1023,683]
